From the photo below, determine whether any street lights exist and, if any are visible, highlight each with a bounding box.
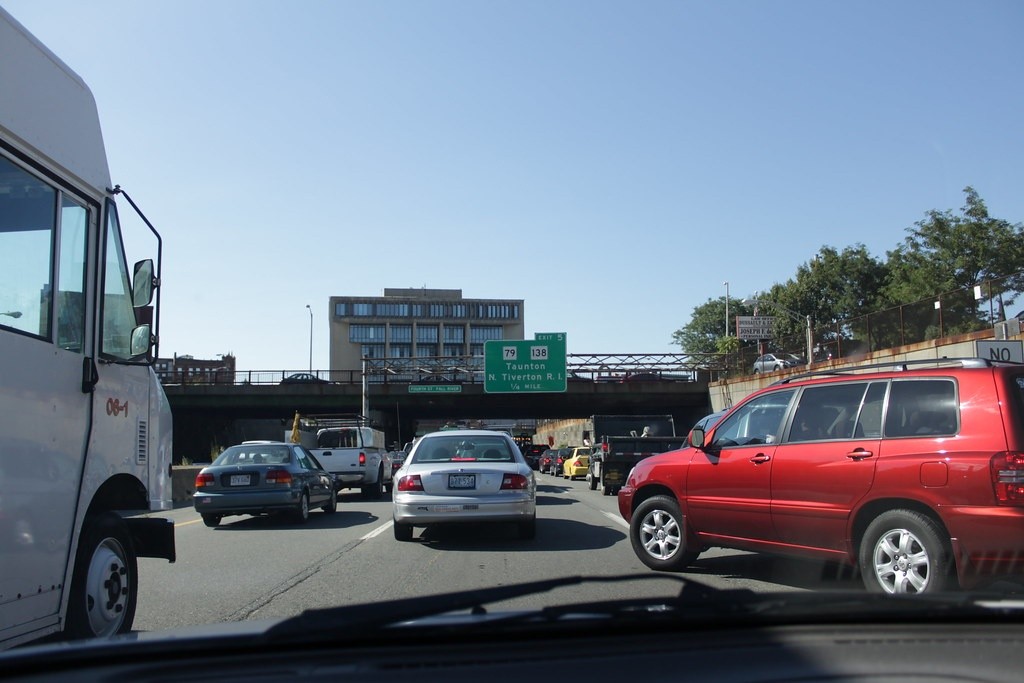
[723,280,729,337]
[741,298,814,366]
[306,305,313,375]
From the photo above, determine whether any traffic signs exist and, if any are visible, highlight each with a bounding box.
[482,332,568,394]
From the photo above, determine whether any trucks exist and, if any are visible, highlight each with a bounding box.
[583,435,688,498]
[1,0,181,661]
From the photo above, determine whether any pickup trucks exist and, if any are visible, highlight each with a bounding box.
[309,424,393,501]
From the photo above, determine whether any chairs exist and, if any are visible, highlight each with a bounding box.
[911,411,940,435]
[831,420,865,439]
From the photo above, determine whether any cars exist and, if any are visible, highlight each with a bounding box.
[192,439,338,528]
[521,443,591,481]
[373,426,542,541]
[418,375,450,384]
[388,450,408,476]
[619,371,676,386]
[280,372,330,385]
[681,400,846,452]
[751,351,804,375]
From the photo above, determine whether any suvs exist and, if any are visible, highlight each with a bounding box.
[615,354,1024,616]
